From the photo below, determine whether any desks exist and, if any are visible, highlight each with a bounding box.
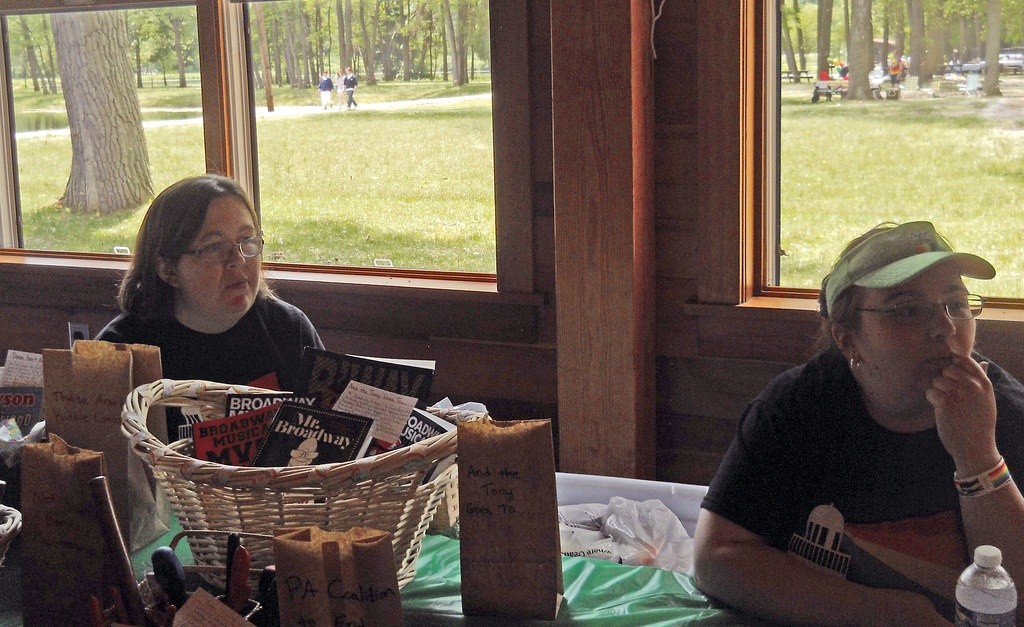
[0,470,1024,627]
[780,70,809,76]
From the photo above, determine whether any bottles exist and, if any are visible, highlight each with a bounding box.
[954,544,1018,627]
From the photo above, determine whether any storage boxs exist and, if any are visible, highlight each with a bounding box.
[885,88,899,99]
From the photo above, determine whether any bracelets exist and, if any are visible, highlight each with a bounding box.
[953,456,1012,500]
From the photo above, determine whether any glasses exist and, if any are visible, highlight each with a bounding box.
[858,293,988,325]
[181,235,265,261]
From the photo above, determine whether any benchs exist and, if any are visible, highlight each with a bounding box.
[999,64,1022,75]
[937,64,964,75]
[811,86,848,103]
[781,76,813,84]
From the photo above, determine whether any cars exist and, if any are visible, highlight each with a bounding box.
[832,46,1023,92]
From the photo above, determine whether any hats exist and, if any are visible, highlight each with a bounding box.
[826,220,995,315]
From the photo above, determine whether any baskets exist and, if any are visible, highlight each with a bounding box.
[122,379,488,594]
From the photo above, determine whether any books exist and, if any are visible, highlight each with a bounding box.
[192,351,448,469]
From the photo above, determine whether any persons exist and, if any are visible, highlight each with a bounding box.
[692,222,1024,627]
[318,66,358,112]
[92,173,328,442]
